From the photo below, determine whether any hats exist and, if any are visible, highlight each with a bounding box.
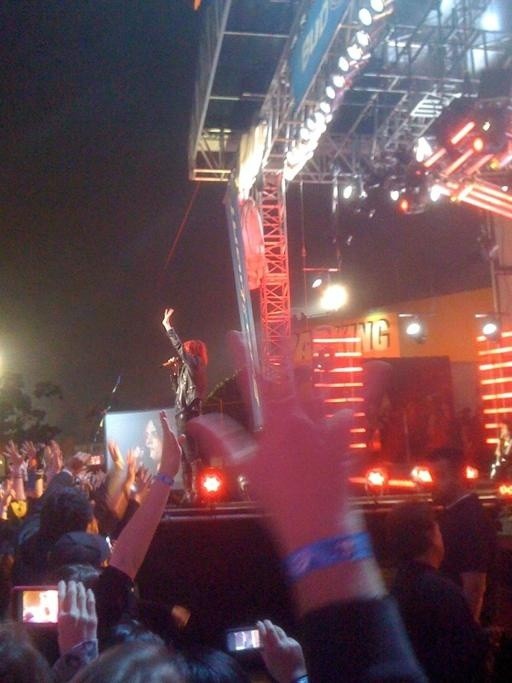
[52,532,111,569]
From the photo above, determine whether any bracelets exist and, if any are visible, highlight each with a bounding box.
[278,532,376,577]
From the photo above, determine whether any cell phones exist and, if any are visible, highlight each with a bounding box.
[85,454,105,467]
[225,626,264,653]
[10,586,59,626]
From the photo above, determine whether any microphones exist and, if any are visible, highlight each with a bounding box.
[158,356,179,368]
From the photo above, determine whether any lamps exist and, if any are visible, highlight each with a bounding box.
[357,8,395,25]
[413,134,448,170]
[412,465,436,494]
[338,53,369,72]
[398,311,427,342]
[470,114,508,153]
[365,470,388,508]
[310,272,325,288]
[475,312,501,338]
[458,465,479,490]
[324,85,348,98]
[346,42,375,60]
[331,70,358,88]
[203,468,225,515]
[341,184,376,205]
[385,175,445,214]
[497,480,512,505]
[282,97,333,180]
[355,20,389,49]
[370,0,395,10]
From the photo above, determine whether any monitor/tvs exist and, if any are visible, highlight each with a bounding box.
[103,407,184,491]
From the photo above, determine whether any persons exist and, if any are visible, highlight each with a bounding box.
[187,326,423,683]
[161,306,210,507]
[387,501,511,683]
[184,616,311,683]
[0,412,183,682]
